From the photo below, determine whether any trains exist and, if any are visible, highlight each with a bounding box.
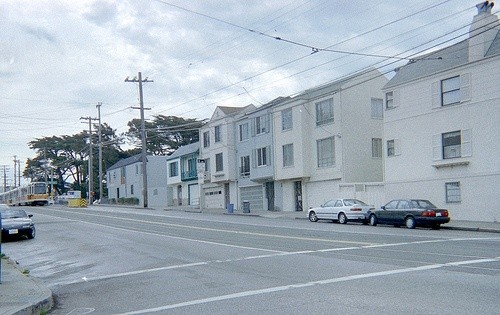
[0,181,48,206]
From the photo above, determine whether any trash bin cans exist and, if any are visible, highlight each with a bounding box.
[227,203,233,214]
[242,200,251,213]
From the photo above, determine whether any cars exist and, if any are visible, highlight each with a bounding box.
[365,198,450,230]
[0,208,35,242]
[307,198,375,224]
